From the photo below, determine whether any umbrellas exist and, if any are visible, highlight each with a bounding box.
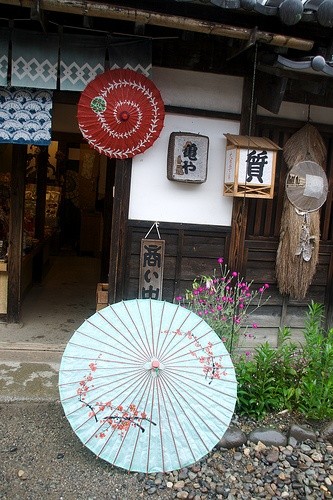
[78,69,166,161]
[54,297,239,475]
